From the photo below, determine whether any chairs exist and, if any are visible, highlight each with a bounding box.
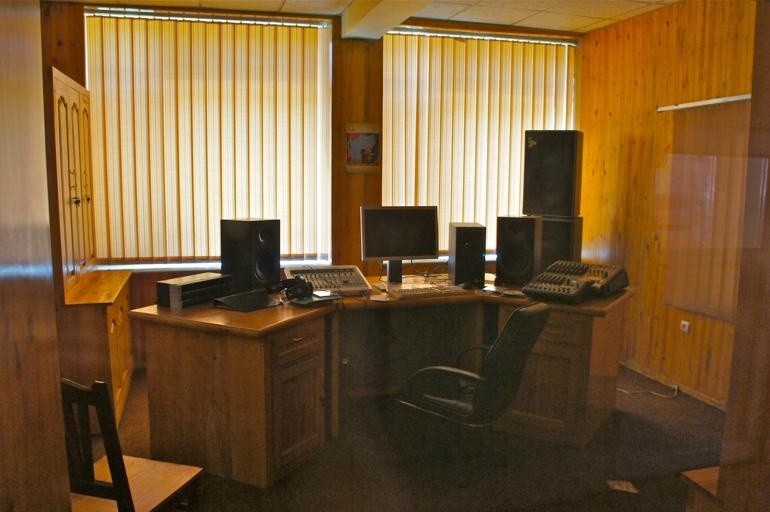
[383,298,553,485]
[60,380,205,512]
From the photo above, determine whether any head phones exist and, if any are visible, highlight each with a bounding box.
[281,275,313,301]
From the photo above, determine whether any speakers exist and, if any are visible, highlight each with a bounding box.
[522,129,582,217]
[494,216,535,285]
[448,222,487,289]
[534,213,583,281]
[220,217,281,290]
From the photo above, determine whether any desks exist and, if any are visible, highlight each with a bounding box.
[128,272,634,493]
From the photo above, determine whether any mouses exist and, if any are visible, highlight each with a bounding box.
[460,281,473,291]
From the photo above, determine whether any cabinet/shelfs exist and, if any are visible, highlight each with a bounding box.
[43,65,133,435]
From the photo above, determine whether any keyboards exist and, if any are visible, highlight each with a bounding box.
[390,283,466,299]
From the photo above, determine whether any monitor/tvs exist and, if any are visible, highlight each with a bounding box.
[359,205,439,284]
